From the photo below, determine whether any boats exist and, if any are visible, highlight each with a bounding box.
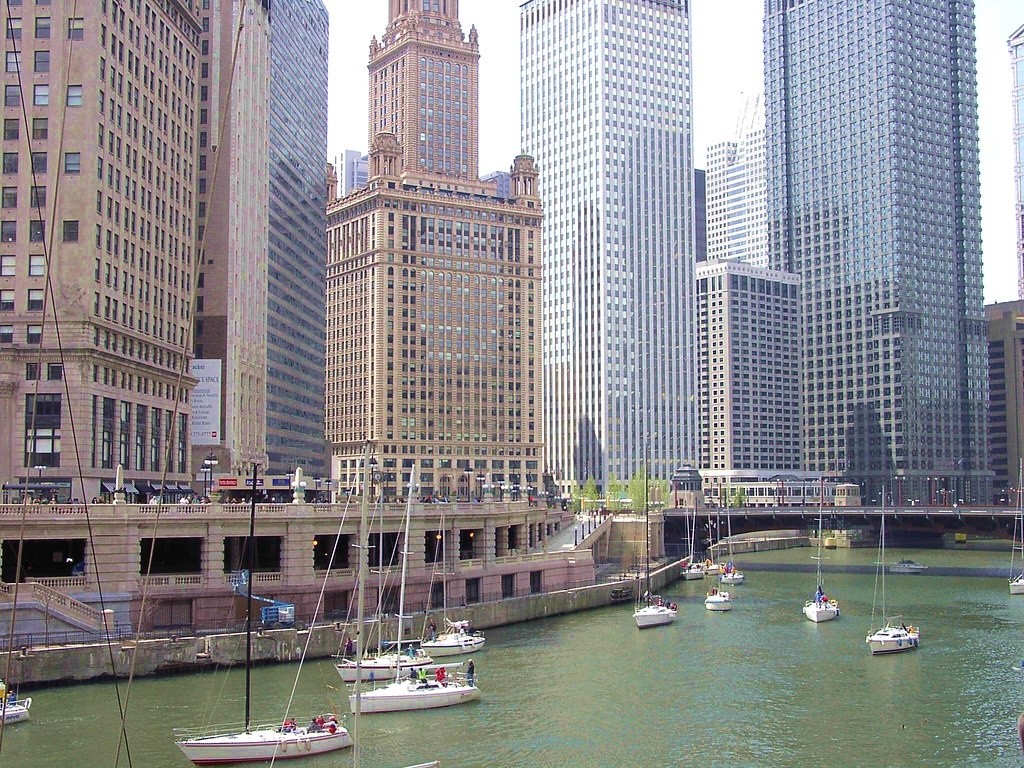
[0,673,35,726]
[887,559,926,576]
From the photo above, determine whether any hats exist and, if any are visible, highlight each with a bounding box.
[468,659,472,662]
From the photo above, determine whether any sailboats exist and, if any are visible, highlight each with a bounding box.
[416,509,486,658]
[705,508,735,612]
[332,472,435,684]
[169,571,353,765]
[865,485,920,655]
[802,502,841,622]
[633,441,677,630]
[1006,458,1024,595]
[680,479,745,585]
[349,464,482,714]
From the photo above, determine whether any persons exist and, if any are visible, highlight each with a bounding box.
[0,678,16,708]
[407,667,426,681]
[814,584,829,605]
[91,494,106,503]
[467,659,475,687]
[238,497,252,505]
[344,492,352,503]
[434,667,446,687]
[344,637,353,661]
[643,557,737,611]
[309,716,338,734]
[425,618,436,642]
[403,643,418,659]
[375,491,484,503]
[180,494,209,505]
[275,718,297,733]
[21,492,83,504]
[149,496,158,504]
[310,495,330,503]
[270,496,277,503]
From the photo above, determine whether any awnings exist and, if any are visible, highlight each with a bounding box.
[102,482,139,494]
[135,485,154,495]
[179,485,192,491]
[165,485,178,490]
[151,483,165,491]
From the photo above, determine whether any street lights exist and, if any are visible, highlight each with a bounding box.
[463,465,474,504]
[369,451,378,503]
[204,452,219,494]
[313,471,320,502]
[285,466,294,503]
[474,467,576,509]
[200,464,211,498]
[324,475,333,503]
[35,465,46,500]
